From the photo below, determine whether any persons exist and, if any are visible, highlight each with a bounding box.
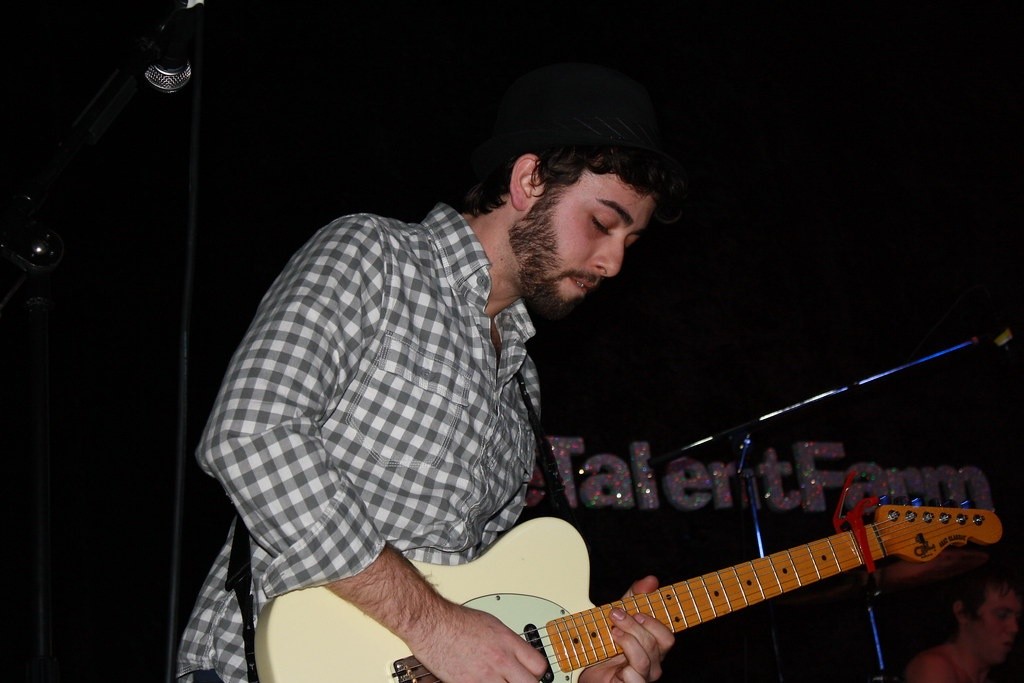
[175,102,677,683]
[902,569,1024,683]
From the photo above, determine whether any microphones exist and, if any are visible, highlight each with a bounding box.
[144,0,204,94]
[991,299,1024,365]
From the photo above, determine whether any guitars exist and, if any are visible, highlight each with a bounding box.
[251,487,1003,683]
